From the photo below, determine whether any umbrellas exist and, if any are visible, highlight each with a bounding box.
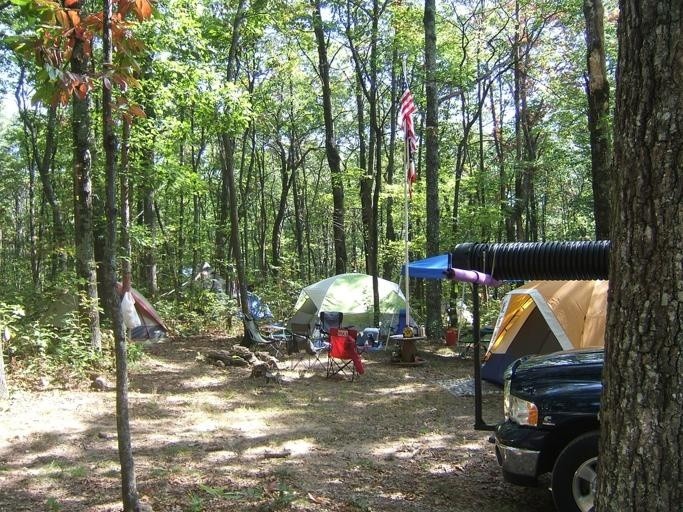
[399,255,466,343]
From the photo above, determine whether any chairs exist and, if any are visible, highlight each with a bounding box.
[227,294,493,380]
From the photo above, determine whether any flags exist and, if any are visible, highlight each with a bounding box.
[396,57,418,194]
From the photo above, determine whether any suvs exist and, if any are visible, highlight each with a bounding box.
[489,344,606,512]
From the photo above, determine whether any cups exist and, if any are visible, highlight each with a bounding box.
[417,328,425,336]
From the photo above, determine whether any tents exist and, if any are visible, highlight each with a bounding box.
[116,280,171,342]
[477,277,608,387]
[284,271,414,345]
